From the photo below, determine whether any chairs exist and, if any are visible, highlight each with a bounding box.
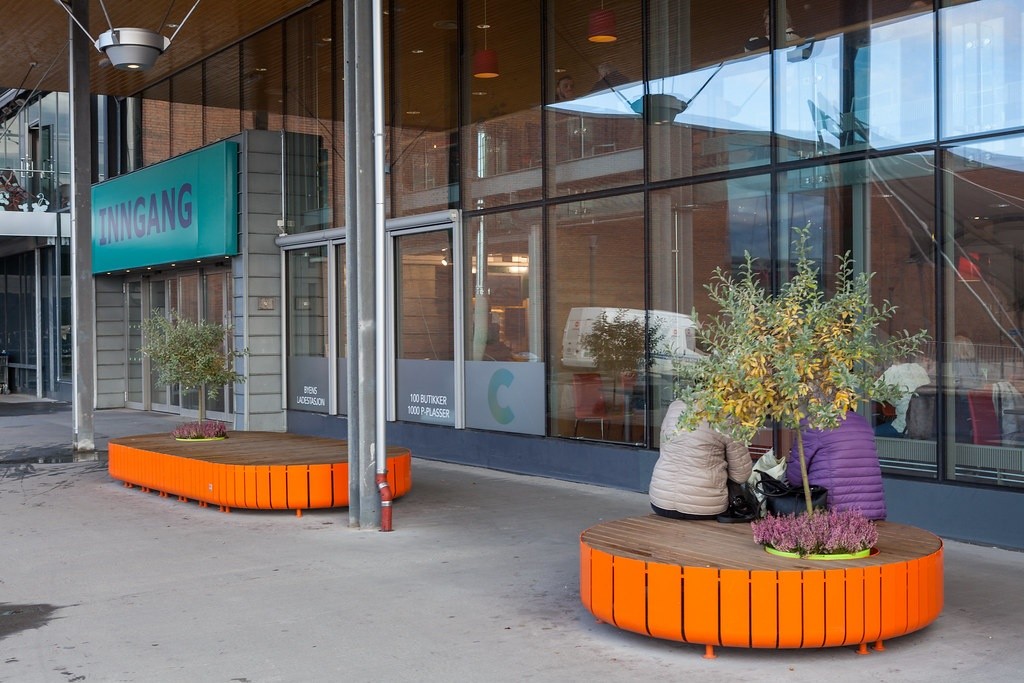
[571,373,625,440]
[905,384,939,441]
[620,370,644,441]
[967,387,1023,449]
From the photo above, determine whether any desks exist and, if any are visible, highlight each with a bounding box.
[1003,408,1024,416]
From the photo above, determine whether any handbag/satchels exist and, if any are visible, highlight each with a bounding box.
[718,478,763,524]
[754,470,828,519]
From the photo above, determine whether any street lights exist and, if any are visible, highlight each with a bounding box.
[585,233,599,307]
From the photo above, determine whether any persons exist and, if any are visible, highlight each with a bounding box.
[647,379,753,519]
[954,336,990,441]
[789,410,887,522]
[873,344,930,437]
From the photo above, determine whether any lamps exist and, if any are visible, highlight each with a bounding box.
[588,0,618,44]
[472,0,501,80]
[53,0,201,73]
[538,1,768,127]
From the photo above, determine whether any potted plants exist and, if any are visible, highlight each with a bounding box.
[135,306,250,440]
[659,219,879,560]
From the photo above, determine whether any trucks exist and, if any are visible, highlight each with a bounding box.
[561,307,718,376]
